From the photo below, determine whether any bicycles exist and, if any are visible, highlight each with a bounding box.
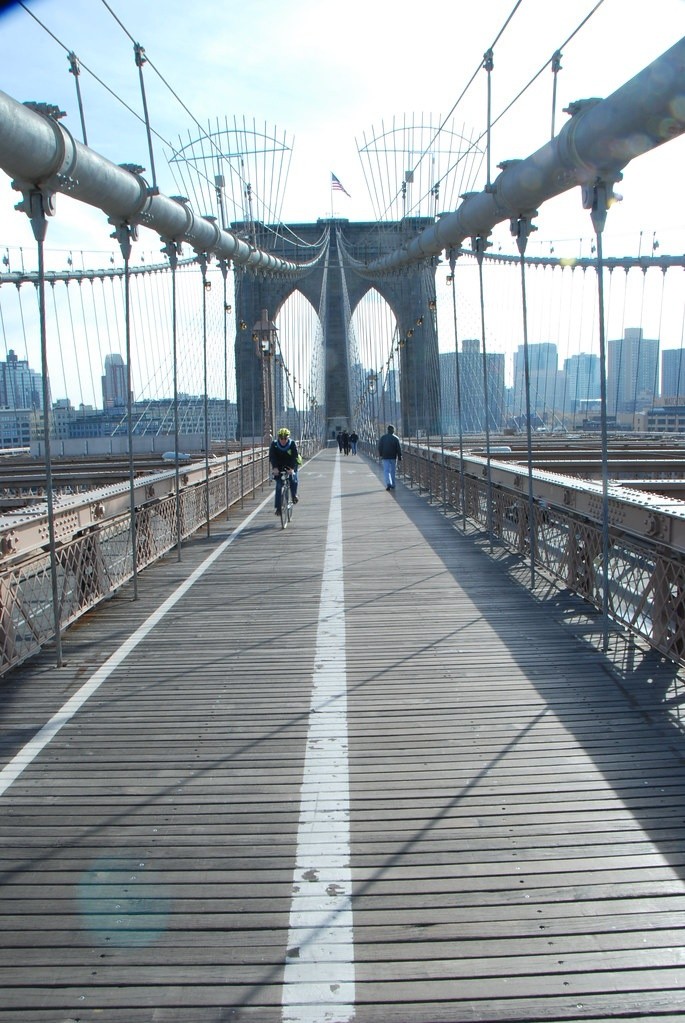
[272,469,297,530]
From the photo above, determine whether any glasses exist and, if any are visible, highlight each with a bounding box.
[278,437,288,440]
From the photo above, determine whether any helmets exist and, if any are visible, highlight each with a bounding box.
[277,428,290,435]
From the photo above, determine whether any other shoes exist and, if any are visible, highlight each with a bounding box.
[275,507,280,515]
[386,485,391,490]
[292,495,298,504]
[391,486,395,488]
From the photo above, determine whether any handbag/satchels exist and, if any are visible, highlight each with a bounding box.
[287,449,302,465]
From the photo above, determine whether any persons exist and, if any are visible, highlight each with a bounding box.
[332,431,335,439]
[342,430,349,456]
[269,428,302,515]
[379,425,402,491]
[349,431,358,455]
[337,432,342,453]
